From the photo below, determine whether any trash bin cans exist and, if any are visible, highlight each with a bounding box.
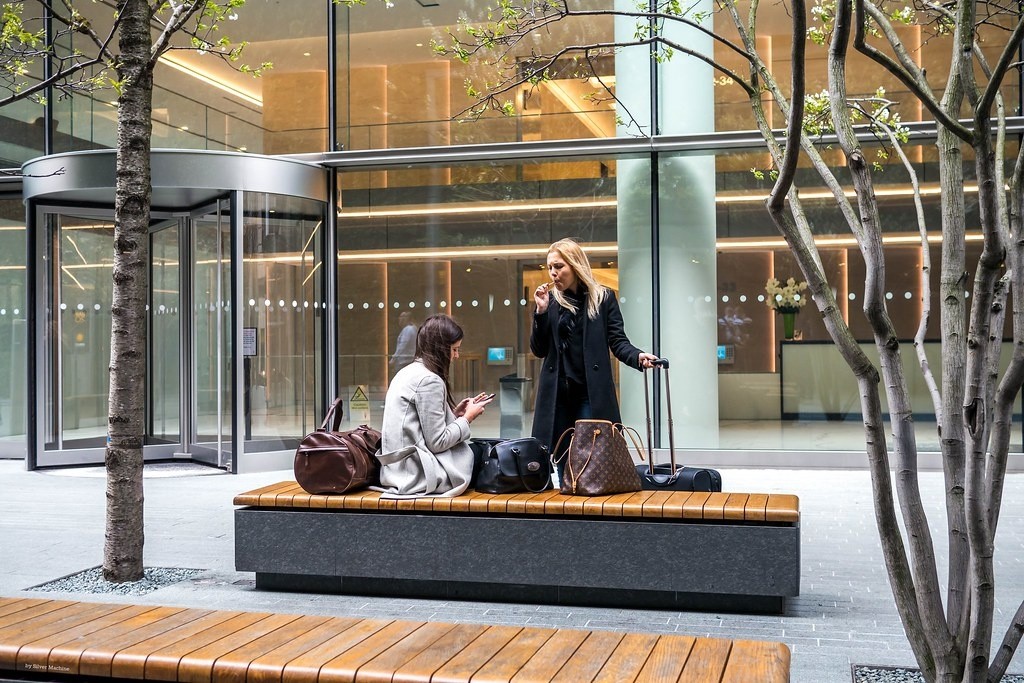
[498,374,524,440]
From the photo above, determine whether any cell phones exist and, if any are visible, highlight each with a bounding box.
[484,393,496,401]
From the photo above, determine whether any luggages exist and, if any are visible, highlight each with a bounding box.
[634,358,722,493]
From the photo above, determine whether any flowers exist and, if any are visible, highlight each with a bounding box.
[766,277,808,314]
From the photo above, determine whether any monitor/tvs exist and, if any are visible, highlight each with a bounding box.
[487,347,505,361]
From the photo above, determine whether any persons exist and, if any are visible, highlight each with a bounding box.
[531,237,663,464]
[375,313,493,499]
[380,312,419,409]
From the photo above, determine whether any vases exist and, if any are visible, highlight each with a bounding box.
[783,313,795,342]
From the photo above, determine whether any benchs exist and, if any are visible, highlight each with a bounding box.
[233,479,801,614]
[1,597,790,683]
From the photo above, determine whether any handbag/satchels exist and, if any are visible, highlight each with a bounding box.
[552,420,645,495]
[293,398,382,495]
[471,438,555,494]
